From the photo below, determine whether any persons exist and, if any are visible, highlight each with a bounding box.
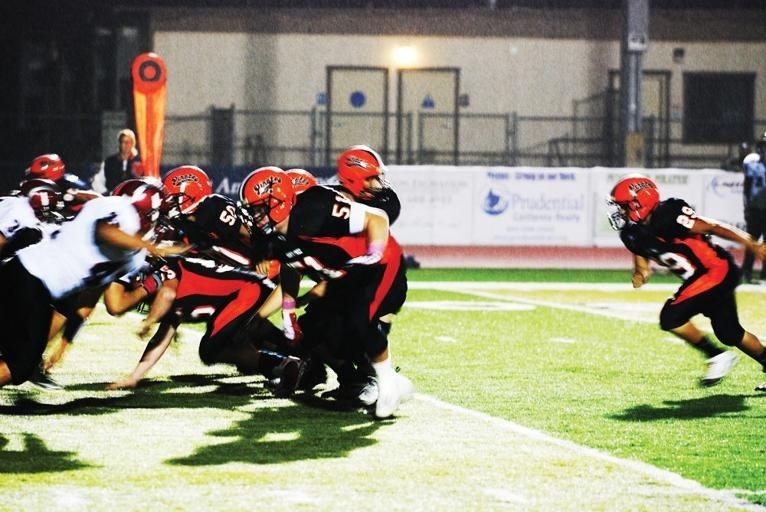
[91,129,141,195]
[236,166,414,419]
[161,165,327,392]
[103,255,308,398]
[0,178,132,375]
[604,174,762,392]
[324,144,400,228]
[0,177,61,260]
[0,179,168,406]
[11,152,65,197]
[740,143,766,284]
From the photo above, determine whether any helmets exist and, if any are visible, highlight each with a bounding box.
[113,180,165,236]
[234,166,296,236]
[286,169,315,195]
[605,178,658,233]
[365,185,401,227]
[28,187,57,219]
[339,144,384,202]
[24,154,66,184]
[163,165,212,218]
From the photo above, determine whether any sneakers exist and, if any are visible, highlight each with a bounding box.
[327,372,354,397]
[700,350,738,386]
[359,366,399,406]
[372,374,413,417]
[756,381,765,391]
[341,372,371,405]
[275,360,298,397]
[294,359,328,391]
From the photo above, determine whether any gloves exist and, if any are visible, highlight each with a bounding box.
[281,308,303,341]
[348,249,384,265]
[145,270,169,294]
